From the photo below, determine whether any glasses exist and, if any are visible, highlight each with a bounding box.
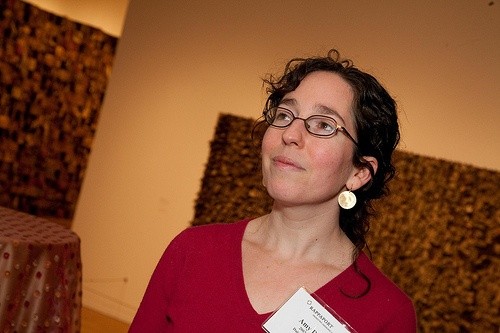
[266,107,359,147]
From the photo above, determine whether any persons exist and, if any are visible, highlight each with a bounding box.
[129,49,416,332]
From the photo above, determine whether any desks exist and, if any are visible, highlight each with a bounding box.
[1,207,82,333]
